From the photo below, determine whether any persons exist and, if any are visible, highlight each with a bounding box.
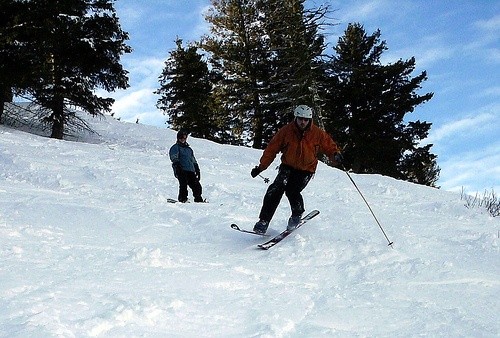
[169,131,204,203]
[251,105,343,234]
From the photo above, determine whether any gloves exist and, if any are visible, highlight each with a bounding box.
[335,154,344,161]
[175,167,184,183]
[193,163,200,181]
[251,165,262,178]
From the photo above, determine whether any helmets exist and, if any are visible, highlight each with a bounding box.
[293,105,313,118]
[177,130,187,138]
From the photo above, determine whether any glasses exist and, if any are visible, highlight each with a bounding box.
[297,117,310,121]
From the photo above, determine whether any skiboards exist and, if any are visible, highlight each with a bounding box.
[167,198,224,206]
[231,210,320,251]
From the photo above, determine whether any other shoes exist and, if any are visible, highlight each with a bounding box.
[194,197,203,202]
[178,197,188,203]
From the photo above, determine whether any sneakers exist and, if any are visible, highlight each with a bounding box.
[288,213,302,226]
[253,220,269,233]
